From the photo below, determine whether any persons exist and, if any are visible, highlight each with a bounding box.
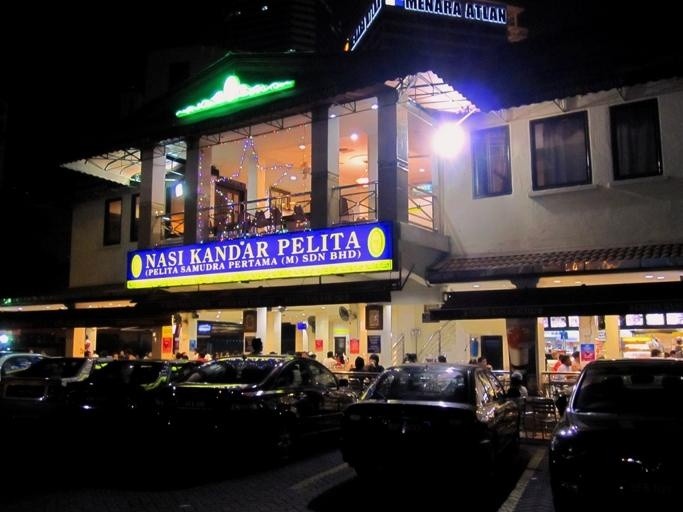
[650,338,683,358]
[249,338,263,356]
[470,357,492,370]
[506,371,530,424]
[291,350,384,384]
[438,355,447,363]
[404,353,417,364]
[174,348,212,363]
[551,350,581,418]
[85,340,152,360]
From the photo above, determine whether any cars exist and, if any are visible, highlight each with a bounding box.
[549,359,683,512]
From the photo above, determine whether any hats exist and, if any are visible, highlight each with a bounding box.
[511,371,522,380]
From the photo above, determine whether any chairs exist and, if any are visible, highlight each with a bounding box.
[523,395,559,440]
